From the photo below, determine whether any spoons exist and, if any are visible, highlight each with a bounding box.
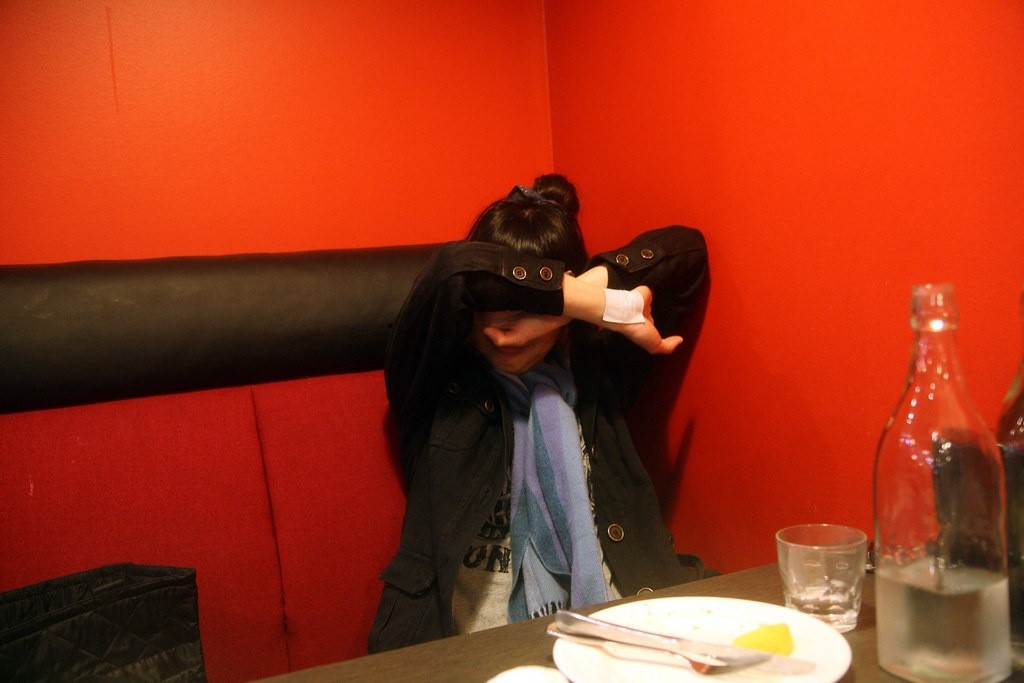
[546,615,769,672]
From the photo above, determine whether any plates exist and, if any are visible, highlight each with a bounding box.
[555,595,856,683]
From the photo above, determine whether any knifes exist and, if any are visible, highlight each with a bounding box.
[558,607,819,674]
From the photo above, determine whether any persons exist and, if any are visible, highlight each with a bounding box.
[367,174,708,655]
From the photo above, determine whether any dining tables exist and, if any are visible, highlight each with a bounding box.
[248,541,1024,683]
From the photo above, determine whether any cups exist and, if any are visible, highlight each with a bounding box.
[777,520,867,634]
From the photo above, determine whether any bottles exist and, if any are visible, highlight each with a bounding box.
[872,281,1017,683]
